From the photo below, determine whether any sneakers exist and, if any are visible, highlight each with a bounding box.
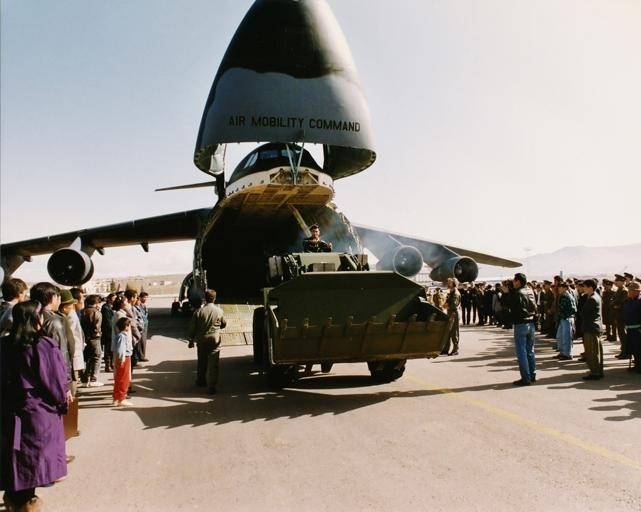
[133,358,149,369]
[513,379,530,385]
[195,380,206,386]
[579,352,585,362]
[106,368,114,372]
[90,382,104,386]
[552,347,572,360]
[206,387,216,393]
[615,353,632,359]
[583,375,600,380]
[448,349,458,356]
[113,387,136,405]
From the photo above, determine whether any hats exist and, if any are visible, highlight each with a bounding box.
[606,273,641,291]
[544,277,575,287]
[59,289,78,305]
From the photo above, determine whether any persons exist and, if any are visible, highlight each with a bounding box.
[424,273,641,387]
[303,224,332,252]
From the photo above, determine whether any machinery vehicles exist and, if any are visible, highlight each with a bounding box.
[250,249,455,389]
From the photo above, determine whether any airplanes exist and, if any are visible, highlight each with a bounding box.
[2,2,523,347]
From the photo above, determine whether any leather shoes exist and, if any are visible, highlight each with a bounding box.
[65,454,75,463]
